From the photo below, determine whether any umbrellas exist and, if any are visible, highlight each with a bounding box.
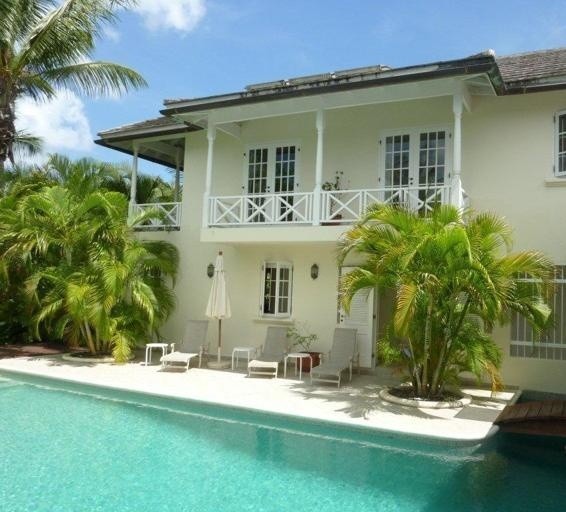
[204,253,232,364]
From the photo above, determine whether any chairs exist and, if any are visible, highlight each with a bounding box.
[310,328,360,389]
[247,326,288,378]
[159,320,209,372]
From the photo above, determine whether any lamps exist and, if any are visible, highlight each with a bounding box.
[311,263,318,279]
[208,264,214,277]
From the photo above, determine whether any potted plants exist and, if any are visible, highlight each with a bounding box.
[322,171,344,226]
[286,327,322,373]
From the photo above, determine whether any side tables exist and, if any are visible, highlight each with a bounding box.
[145,343,168,367]
[231,346,255,370]
[284,352,313,380]
